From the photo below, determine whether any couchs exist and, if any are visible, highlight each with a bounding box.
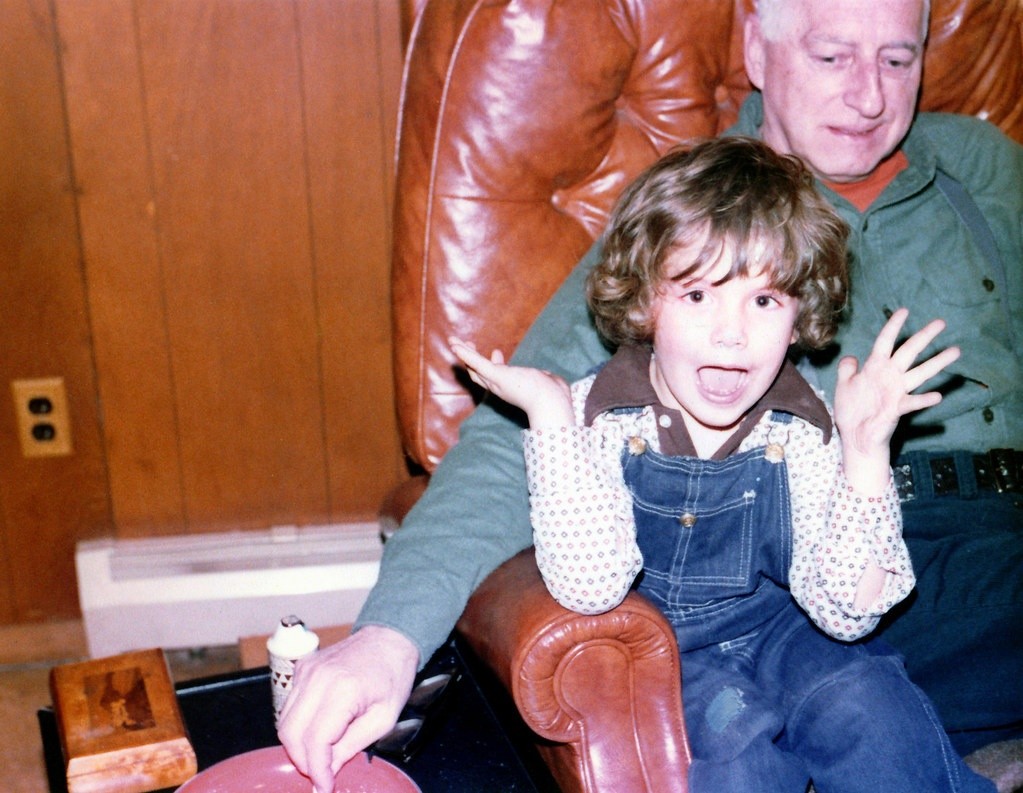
[391,0,1023,793]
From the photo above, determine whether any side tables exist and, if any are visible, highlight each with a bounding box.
[38,624,560,793]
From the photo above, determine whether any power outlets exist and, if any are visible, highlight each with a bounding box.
[16,378,71,457]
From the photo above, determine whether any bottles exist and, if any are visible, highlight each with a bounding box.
[267,616,320,731]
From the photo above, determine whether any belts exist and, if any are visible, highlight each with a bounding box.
[894,447,1022,499]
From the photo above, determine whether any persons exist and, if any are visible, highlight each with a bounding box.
[448,136,1000,792]
[277,1,1023,793]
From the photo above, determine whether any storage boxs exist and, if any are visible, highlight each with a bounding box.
[51,650,200,793]
